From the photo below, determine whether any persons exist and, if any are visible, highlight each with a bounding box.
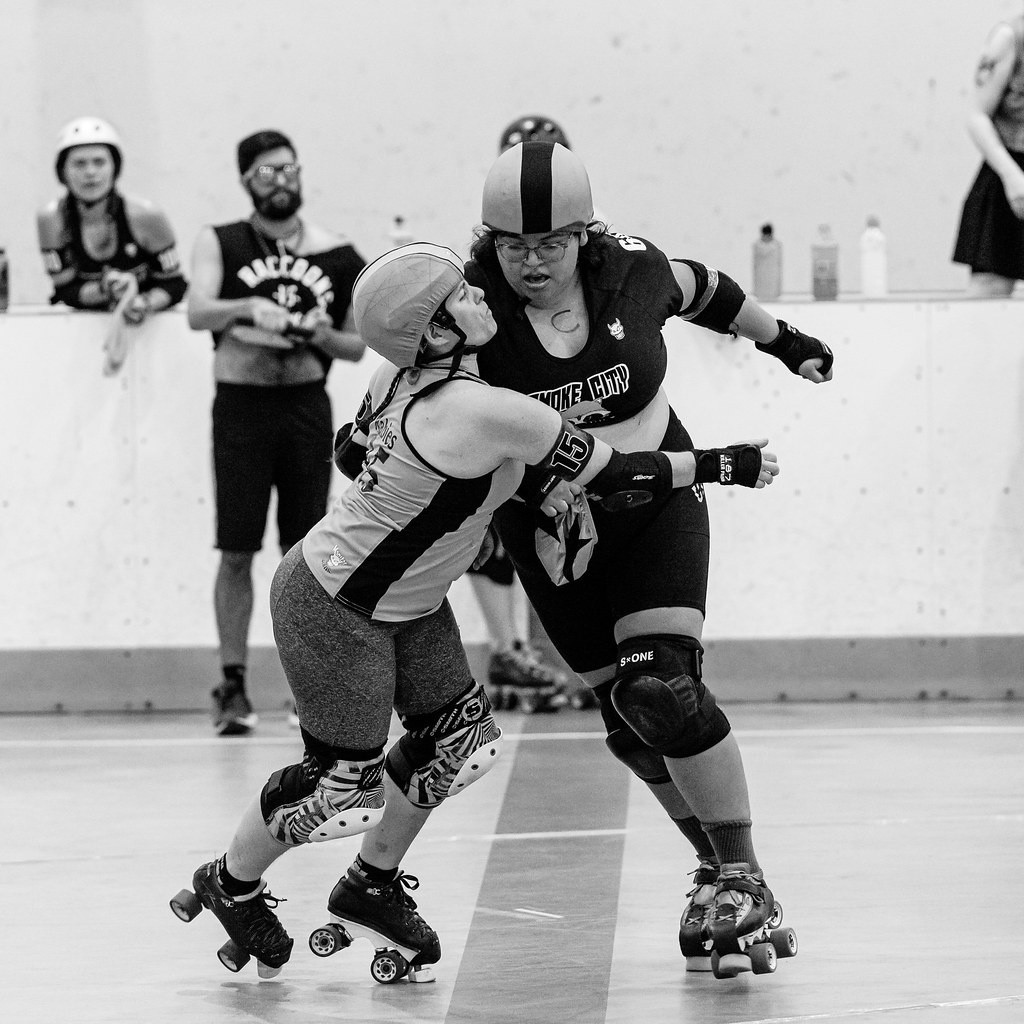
[952,11,1024,302]
[187,129,367,741]
[33,114,191,326]
[463,115,596,715]
[169,243,780,985]
[463,142,835,981]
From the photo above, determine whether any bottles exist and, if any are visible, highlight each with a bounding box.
[859,214,889,299]
[753,226,783,302]
[812,225,839,301]
[0,248,9,310]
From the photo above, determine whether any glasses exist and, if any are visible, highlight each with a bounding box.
[495,232,573,262]
[246,163,302,186]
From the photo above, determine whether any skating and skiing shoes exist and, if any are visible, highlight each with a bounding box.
[308,853,441,985]
[679,854,723,972]
[169,858,294,978]
[710,862,798,980]
[484,641,566,715]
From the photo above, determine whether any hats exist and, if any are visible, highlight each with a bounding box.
[237,131,295,174]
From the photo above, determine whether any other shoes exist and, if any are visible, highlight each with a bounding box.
[210,676,258,736]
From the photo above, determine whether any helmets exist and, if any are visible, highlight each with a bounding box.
[501,117,571,154]
[355,242,466,372]
[54,116,126,185]
[481,141,594,235]
